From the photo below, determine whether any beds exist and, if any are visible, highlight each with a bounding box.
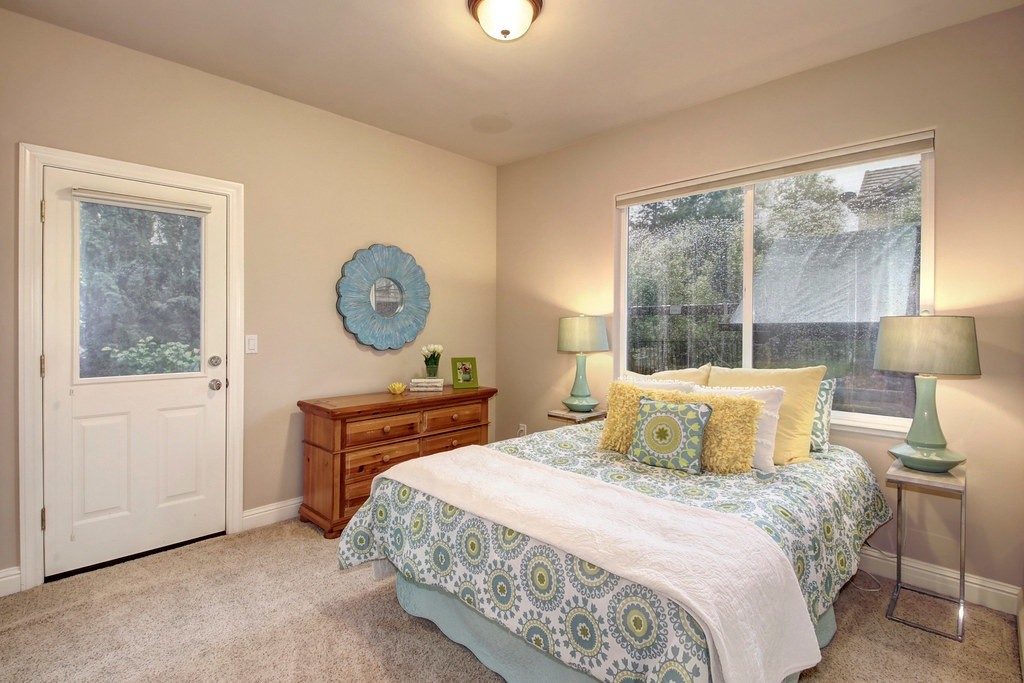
[340,420,894,683]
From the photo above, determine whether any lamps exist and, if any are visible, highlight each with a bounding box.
[467,0,543,41]
[870,308,982,473]
[557,313,610,412]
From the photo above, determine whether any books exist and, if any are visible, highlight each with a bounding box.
[409,378,444,392]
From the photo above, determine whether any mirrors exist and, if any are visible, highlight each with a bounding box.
[369,276,405,318]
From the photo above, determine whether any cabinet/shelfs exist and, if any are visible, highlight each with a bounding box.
[547,409,608,425]
[885,456,969,643]
[297,383,500,538]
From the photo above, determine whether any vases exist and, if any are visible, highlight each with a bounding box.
[425,365,439,379]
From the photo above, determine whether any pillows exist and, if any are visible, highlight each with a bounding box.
[808,376,837,453]
[709,364,828,463]
[624,362,713,388]
[626,395,712,477]
[619,378,785,474]
[598,380,766,472]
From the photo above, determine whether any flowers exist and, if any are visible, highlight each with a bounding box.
[420,343,444,376]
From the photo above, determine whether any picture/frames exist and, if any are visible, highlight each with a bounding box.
[451,357,480,388]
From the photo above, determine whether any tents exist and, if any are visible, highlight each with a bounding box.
[730,223,921,334]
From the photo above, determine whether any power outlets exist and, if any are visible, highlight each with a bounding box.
[519,424,526,436]
